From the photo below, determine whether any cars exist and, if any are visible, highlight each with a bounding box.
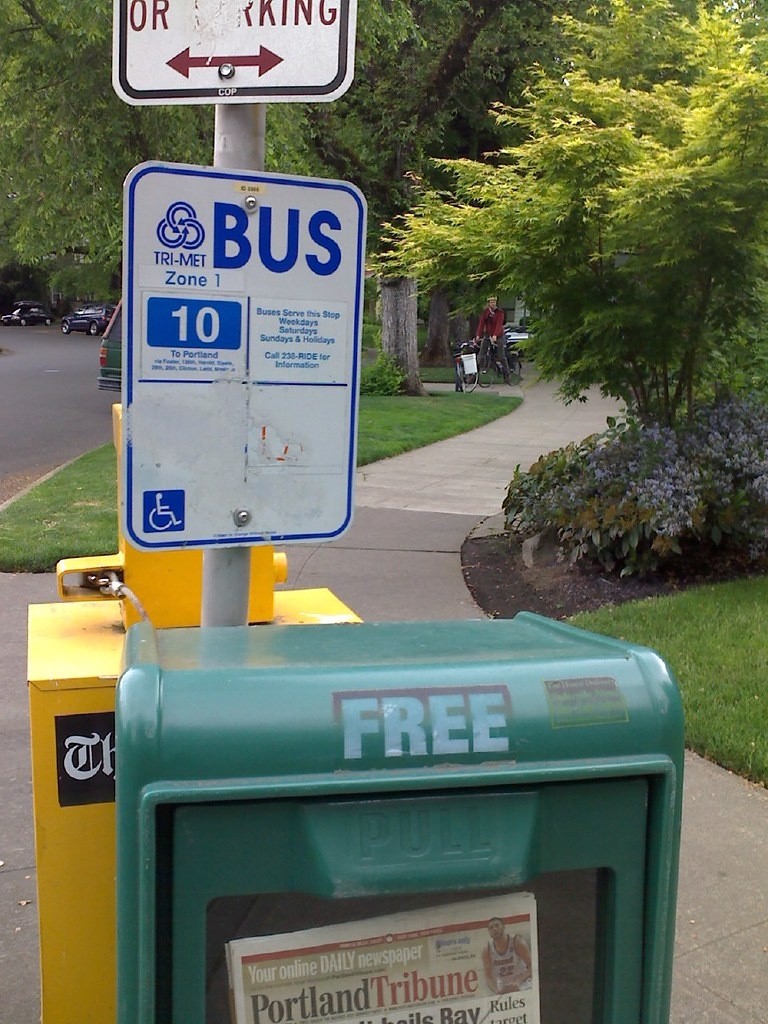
[0,307,55,327]
[9,301,44,312]
[504,326,535,351]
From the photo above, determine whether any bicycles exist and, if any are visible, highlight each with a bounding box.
[451,333,522,392]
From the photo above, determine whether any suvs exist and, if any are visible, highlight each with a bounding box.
[97,297,123,392]
[60,302,117,336]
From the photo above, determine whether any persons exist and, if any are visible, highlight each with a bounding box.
[466,296,511,383]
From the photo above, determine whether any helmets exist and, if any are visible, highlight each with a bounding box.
[487,296,497,302]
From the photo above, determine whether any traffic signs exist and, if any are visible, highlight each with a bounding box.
[109,0,357,108]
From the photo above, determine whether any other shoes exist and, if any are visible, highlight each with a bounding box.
[466,376,479,383]
[504,374,511,383]
